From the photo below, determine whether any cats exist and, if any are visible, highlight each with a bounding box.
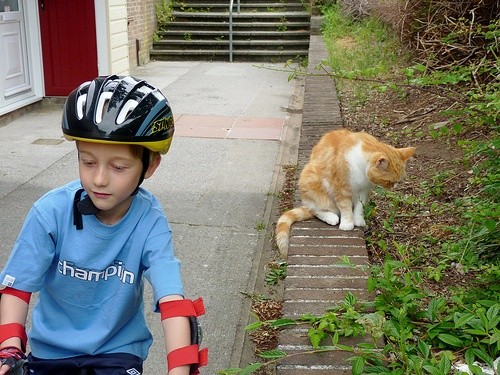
[275,129,416,261]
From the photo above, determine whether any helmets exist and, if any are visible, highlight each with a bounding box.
[61,75,174,156]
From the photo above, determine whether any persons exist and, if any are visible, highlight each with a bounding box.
[0,74,191,375]
